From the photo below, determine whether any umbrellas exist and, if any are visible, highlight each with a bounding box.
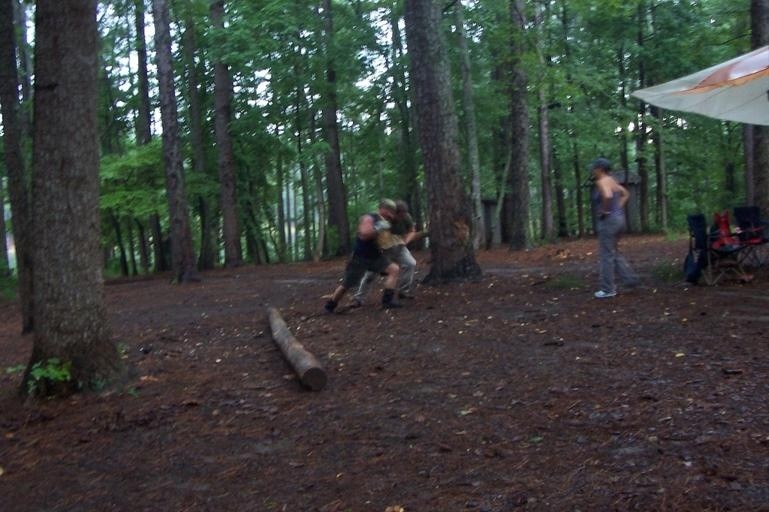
[632,40,769,128]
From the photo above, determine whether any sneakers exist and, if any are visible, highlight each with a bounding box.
[324,298,338,314]
[345,297,360,310]
[595,290,616,298]
[382,300,402,308]
[401,290,416,298]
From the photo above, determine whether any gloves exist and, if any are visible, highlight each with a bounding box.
[373,219,391,232]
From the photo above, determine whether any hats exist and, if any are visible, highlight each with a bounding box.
[383,198,397,211]
[395,200,408,212]
[594,159,611,169]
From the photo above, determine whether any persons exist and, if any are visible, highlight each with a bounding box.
[590,158,640,299]
[345,199,417,309]
[325,198,403,312]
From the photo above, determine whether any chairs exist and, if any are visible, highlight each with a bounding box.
[682,206,769,287]
